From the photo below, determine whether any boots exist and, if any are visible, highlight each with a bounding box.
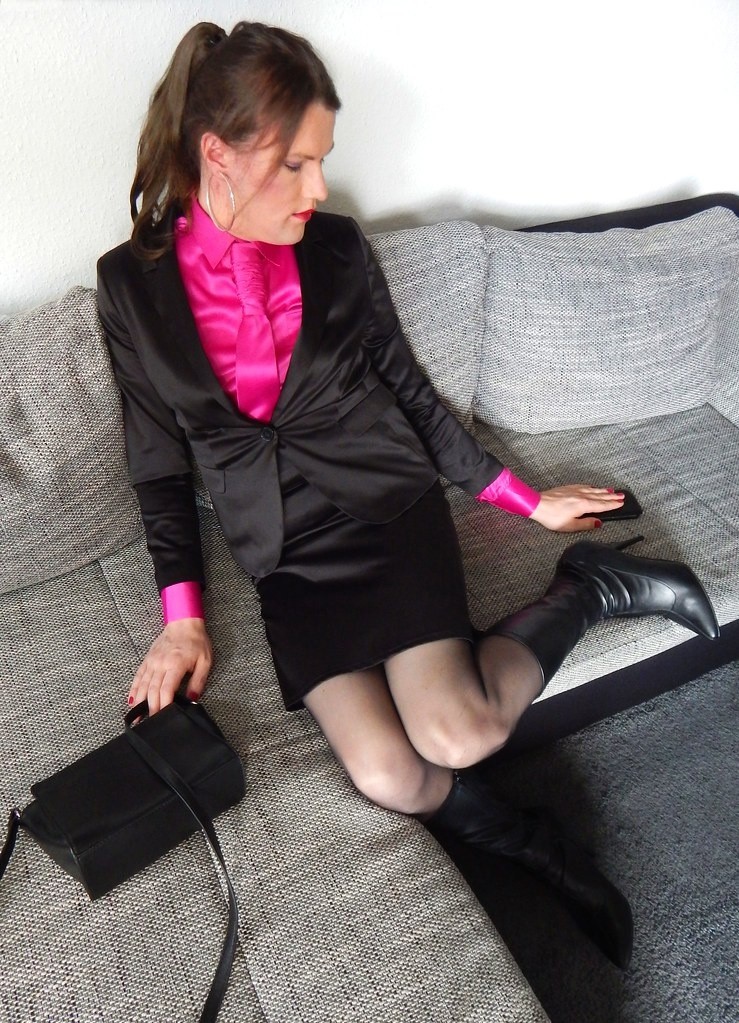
[430,769,632,971]
[486,536,721,697]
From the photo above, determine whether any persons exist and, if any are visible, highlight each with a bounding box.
[96,18,723,974]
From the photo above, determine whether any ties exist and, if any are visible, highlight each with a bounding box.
[232,242,280,424]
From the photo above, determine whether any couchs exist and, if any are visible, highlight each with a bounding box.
[1,193,738,1023]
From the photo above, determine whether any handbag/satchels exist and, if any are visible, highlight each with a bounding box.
[0,698,245,1023]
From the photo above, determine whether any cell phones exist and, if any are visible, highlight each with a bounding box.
[576,489,643,521]
